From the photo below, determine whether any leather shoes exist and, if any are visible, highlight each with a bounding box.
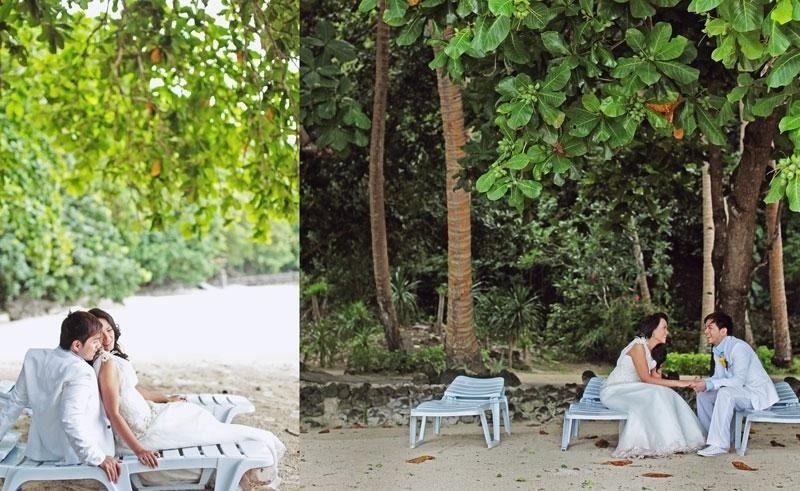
[697,445,729,457]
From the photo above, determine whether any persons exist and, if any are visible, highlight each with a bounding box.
[694,311,780,457]
[598,311,705,457]
[0,311,122,483]
[88,307,288,491]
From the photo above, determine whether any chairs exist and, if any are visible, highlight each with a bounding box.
[408,376,800,458]
[0,381,278,491]
